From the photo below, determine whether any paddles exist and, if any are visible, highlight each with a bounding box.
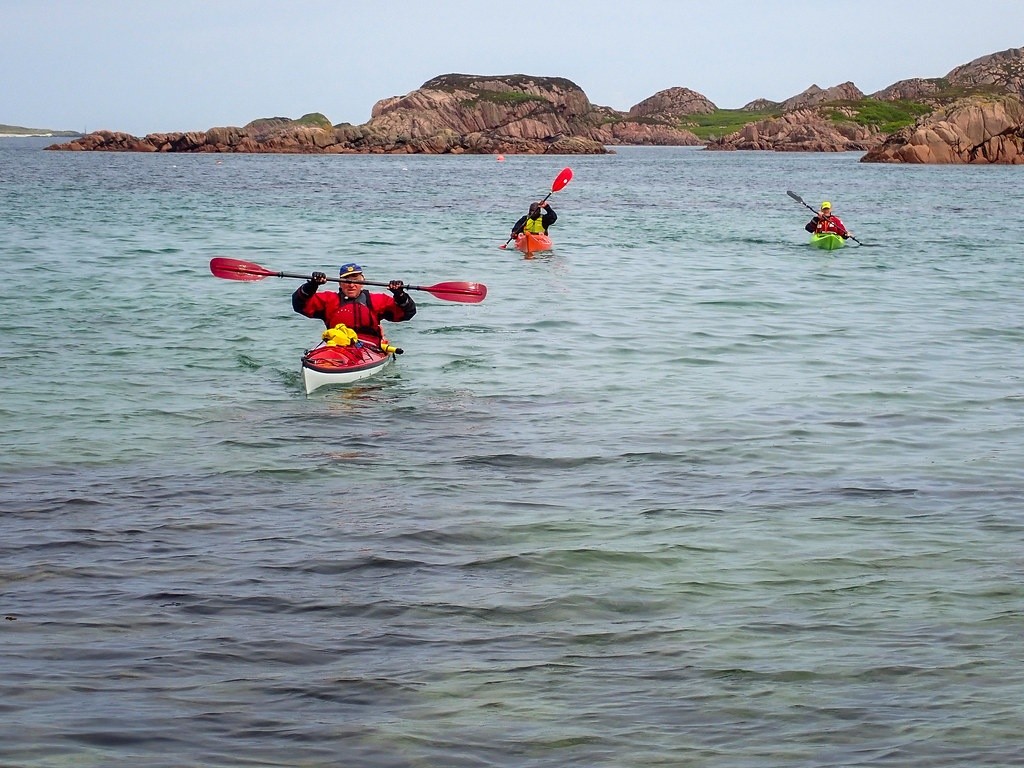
[209,257,487,304]
[499,167,574,249]
[786,190,864,245]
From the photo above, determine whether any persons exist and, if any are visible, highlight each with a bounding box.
[292,263,416,345]
[805,201,851,240]
[511,201,557,240]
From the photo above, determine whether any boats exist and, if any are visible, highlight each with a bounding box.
[515,231,553,259]
[810,232,845,250]
[301,325,395,396]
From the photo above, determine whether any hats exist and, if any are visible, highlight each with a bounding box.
[530,202,541,211]
[821,201,831,210]
[339,263,362,277]
